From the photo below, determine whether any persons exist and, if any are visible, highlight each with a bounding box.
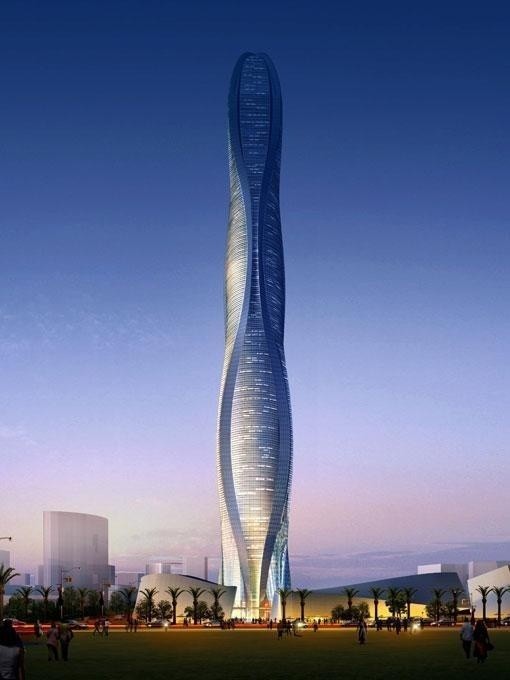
[252,617,292,641]
[421,618,424,630]
[460,618,473,659]
[182,617,192,628]
[125,616,138,633]
[92,617,112,640]
[313,617,336,632]
[374,616,411,635]
[220,617,244,630]
[481,617,502,630]
[472,620,490,664]
[356,616,368,644]
[0,615,74,680]
[164,619,169,632]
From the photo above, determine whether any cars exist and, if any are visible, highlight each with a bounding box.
[430,618,457,628]
[3,618,26,626]
[500,617,510,626]
[66,619,89,630]
